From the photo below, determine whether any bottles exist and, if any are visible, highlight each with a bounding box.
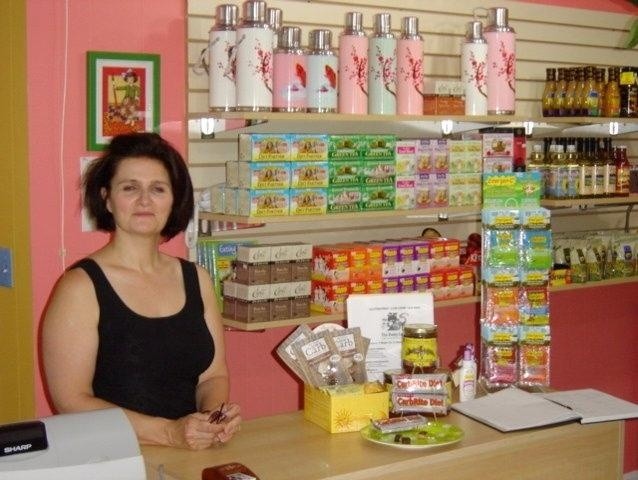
[545,144,556,199]
[591,137,604,199]
[549,144,569,200]
[604,137,616,198]
[565,145,580,200]
[564,67,578,117]
[553,67,568,117]
[579,65,603,117]
[576,141,595,200]
[620,66,638,118]
[600,67,621,117]
[573,67,586,117]
[401,324,439,375]
[616,145,631,198]
[526,144,545,200]
[542,68,557,118]
[458,349,478,403]
[595,67,606,97]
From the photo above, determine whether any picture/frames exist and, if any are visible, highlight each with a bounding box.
[85,51,160,155]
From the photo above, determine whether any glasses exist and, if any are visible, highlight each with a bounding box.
[207,400,227,424]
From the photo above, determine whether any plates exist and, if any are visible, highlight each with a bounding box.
[359,420,465,452]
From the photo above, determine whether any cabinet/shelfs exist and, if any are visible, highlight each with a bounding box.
[186,112,637,333]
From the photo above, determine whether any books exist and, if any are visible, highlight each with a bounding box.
[450,388,638,433]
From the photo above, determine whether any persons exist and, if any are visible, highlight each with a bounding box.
[41,132,243,453]
[111,71,140,126]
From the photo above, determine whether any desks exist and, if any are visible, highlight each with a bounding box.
[139,390,626,480]
